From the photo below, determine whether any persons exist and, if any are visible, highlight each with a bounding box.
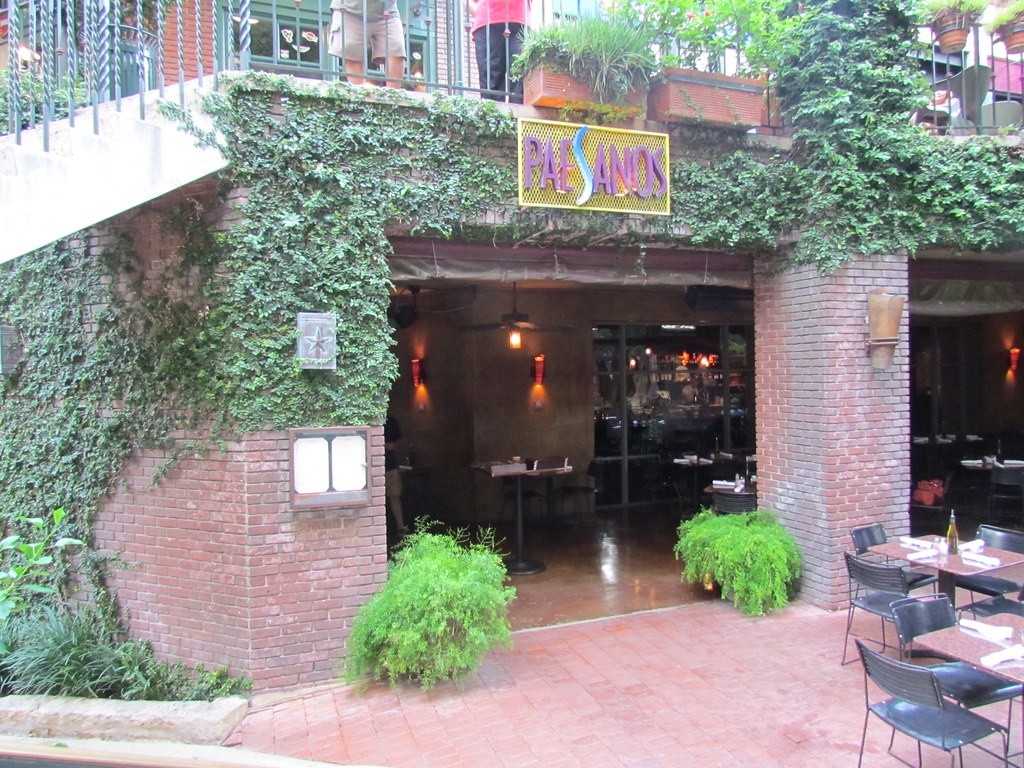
[462,0,533,104]
[328,0,406,89]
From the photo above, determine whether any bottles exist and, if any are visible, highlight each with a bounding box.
[946,515,958,554]
[996,439,1004,465]
[745,456,750,488]
[714,437,720,462]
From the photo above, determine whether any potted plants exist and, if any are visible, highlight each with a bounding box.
[919,0,990,55]
[353,512,518,685]
[983,0,1024,54]
[728,334,747,367]
[506,7,658,119]
[675,508,803,617]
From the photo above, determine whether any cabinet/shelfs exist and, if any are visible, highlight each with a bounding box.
[593,324,755,411]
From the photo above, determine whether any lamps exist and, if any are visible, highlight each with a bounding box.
[863,288,906,369]
[510,326,522,350]
[529,353,549,401]
[1004,346,1020,370]
[411,358,429,406]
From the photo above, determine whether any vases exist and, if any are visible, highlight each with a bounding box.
[647,67,767,131]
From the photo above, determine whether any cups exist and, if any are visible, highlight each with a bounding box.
[524,458,535,471]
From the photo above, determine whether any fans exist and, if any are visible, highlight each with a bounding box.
[456,282,578,335]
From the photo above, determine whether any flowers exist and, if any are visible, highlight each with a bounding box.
[669,11,722,70]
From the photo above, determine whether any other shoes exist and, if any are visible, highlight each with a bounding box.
[397,526,411,535]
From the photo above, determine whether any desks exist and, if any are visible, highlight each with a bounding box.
[681,460,747,519]
[479,470,584,574]
[964,464,993,470]
[913,439,985,462]
[913,613,1024,684]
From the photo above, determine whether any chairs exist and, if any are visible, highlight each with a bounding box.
[915,64,993,135]
[889,593,1024,768]
[956,595,1024,621]
[988,460,1024,523]
[841,551,907,665]
[499,475,534,520]
[954,524,1024,599]
[911,475,952,535]
[850,522,939,600]
[547,477,600,532]
[855,639,1008,768]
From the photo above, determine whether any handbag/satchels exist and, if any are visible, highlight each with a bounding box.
[914,479,944,505]
